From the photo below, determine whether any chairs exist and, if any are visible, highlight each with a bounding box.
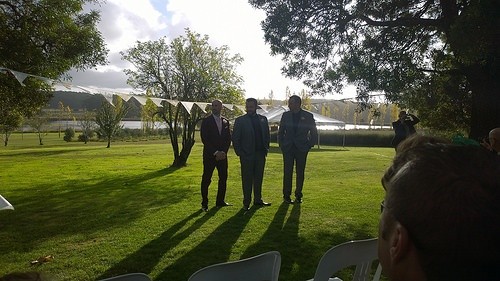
[189,251,281,281]
[307,235,384,281]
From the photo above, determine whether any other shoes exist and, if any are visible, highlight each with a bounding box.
[254,201,272,206]
[296,197,302,203]
[243,205,250,211]
[216,201,233,207]
[283,196,294,203]
[202,206,208,212]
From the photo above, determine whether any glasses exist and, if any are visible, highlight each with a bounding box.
[380,200,402,225]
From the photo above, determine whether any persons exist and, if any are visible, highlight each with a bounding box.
[378,127,500,281]
[277,95,320,203]
[392,110,420,153]
[200,100,233,212]
[232,98,272,210]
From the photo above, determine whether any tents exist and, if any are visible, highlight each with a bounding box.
[256,105,346,150]
